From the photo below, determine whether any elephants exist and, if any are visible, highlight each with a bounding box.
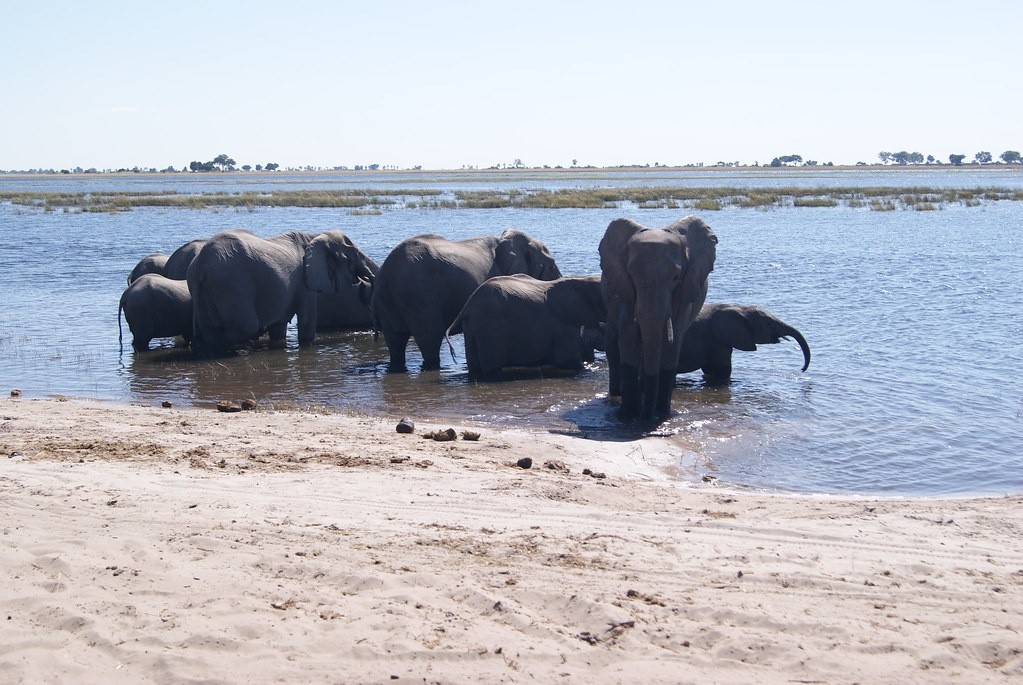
[677,303,811,379]
[597,214,719,419]
[118,229,607,374]
